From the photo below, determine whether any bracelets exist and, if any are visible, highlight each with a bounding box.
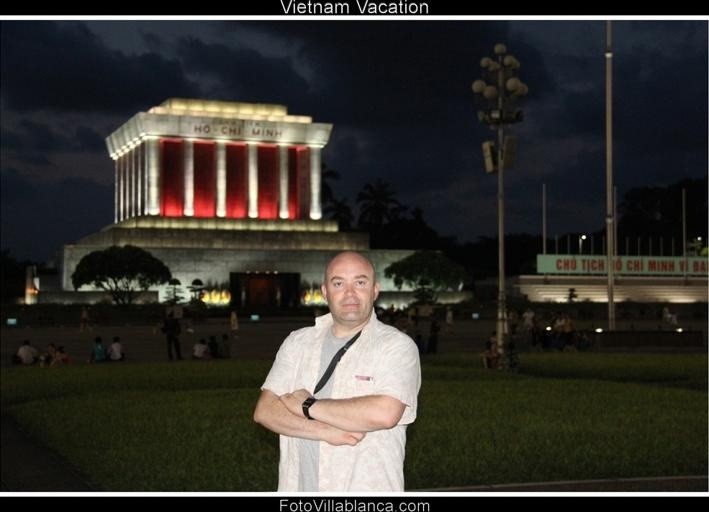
[301,397,317,420]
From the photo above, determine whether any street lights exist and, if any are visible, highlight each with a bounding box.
[471,42,528,358]
[578,234,588,254]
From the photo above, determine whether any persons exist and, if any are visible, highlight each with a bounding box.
[486,307,597,355]
[18,340,76,369]
[252,251,423,493]
[160,310,235,360]
[80,304,92,334]
[86,335,128,362]
[374,302,454,355]
